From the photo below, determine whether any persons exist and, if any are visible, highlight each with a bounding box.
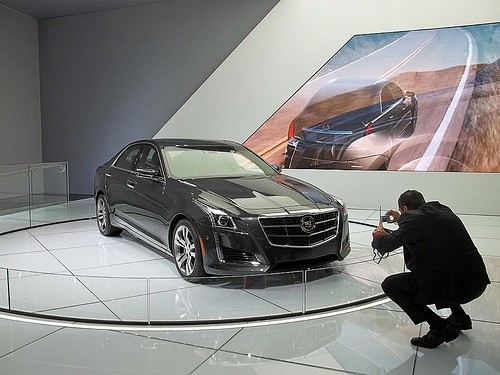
[372,190,491,348]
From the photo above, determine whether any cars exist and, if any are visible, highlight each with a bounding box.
[287,76,418,171]
[92,138,351,284]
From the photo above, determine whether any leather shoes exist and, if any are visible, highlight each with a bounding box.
[445,313,473,330]
[411,320,459,349]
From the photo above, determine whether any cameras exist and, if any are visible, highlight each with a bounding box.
[380,216,390,222]
